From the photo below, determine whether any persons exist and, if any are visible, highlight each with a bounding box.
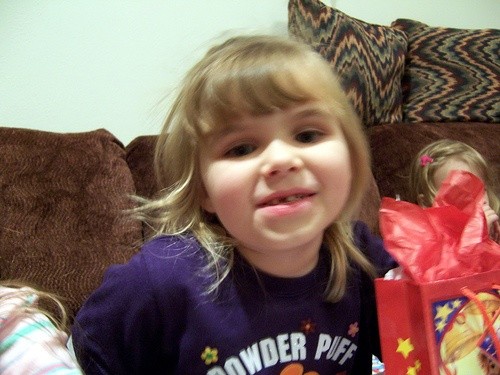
[63,32,401,375]
[407,137,500,245]
[0,281,84,375]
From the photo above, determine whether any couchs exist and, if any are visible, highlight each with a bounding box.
[0,120,500,375]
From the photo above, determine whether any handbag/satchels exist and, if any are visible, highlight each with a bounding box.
[372,269,500,375]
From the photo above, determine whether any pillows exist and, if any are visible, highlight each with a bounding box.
[390,18,500,124]
[287,0,409,129]
